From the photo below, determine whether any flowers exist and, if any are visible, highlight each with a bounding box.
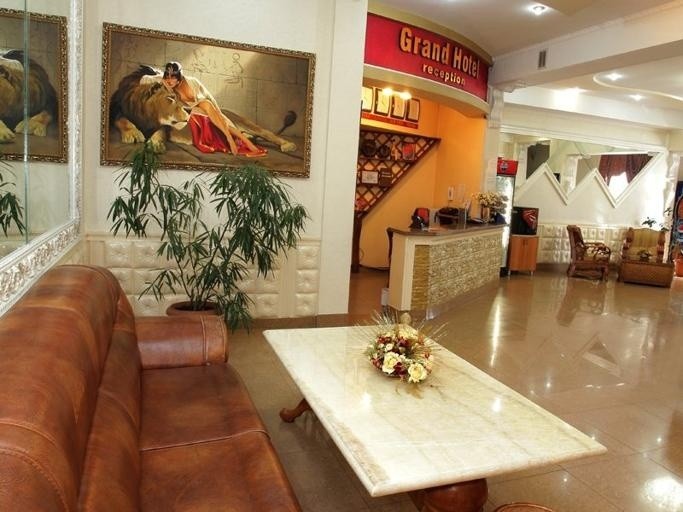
[350,305,450,385]
[471,191,498,208]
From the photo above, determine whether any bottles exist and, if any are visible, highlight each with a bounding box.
[390,139,397,160]
[670,189,683,262]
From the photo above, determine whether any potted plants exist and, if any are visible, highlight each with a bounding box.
[105,141,312,336]
[642,206,683,277]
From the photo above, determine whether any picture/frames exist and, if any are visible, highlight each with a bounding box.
[0,8,68,165]
[98,21,317,178]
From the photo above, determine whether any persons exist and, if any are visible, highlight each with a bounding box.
[139,61,268,158]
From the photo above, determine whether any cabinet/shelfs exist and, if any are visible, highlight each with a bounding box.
[350,124,441,274]
[505,234,539,276]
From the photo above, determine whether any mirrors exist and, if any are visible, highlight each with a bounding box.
[0,0,85,317]
[498,123,667,210]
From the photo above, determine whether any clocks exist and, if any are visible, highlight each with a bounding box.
[360,85,421,122]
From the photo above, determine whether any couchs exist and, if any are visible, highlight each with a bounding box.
[0,263,302,512]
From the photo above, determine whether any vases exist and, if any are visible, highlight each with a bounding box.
[481,206,489,223]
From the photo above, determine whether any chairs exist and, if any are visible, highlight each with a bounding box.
[620,227,665,263]
[566,225,610,282]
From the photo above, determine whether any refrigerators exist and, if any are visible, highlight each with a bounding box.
[496,157,519,278]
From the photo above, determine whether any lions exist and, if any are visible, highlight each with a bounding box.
[0,47,58,145]
[109,64,298,155]
